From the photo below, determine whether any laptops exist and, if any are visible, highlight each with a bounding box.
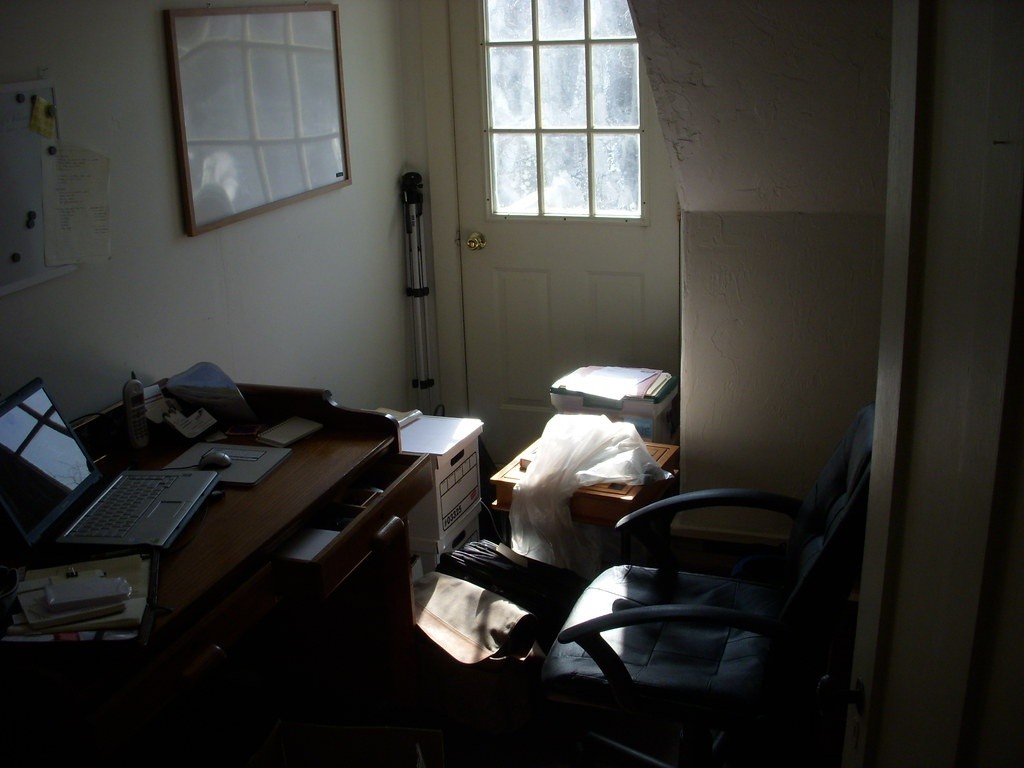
[0,376,219,561]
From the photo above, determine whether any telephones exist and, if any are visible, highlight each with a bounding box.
[122,370,153,471]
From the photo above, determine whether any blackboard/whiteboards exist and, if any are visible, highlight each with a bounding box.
[0,77,79,298]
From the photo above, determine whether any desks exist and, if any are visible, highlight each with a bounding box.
[491,434,683,565]
[0,375,439,768]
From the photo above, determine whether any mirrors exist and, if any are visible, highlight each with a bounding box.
[162,6,353,237]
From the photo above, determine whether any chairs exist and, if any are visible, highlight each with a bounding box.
[538,404,875,768]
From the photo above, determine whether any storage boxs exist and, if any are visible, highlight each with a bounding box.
[550,374,677,444]
[255,717,446,767]
[377,405,483,583]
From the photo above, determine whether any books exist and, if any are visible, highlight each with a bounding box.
[7,554,151,635]
[256,416,323,448]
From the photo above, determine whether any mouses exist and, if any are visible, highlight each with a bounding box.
[198,450,231,471]
